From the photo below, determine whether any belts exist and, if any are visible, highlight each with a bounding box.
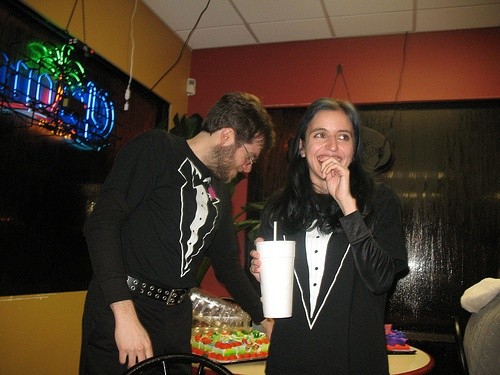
[127,276,195,306]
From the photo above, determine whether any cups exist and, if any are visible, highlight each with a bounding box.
[384,324,392,334]
[255,240,296,318]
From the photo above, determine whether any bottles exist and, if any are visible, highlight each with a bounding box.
[189,288,254,335]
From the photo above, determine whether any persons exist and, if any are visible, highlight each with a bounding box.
[247,97,410,375]
[77,91,277,375]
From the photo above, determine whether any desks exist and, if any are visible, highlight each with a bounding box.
[191,345,436,375]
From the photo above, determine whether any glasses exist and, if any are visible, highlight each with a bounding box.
[238,137,257,165]
